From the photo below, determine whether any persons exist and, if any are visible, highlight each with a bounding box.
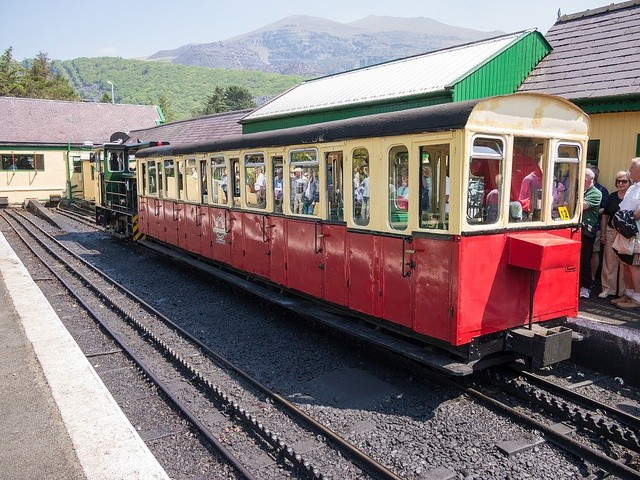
[485,174,501,204]
[599,170,633,298]
[610,158,640,310]
[579,169,602,299]
[585,164,609,288]
[518,153,559,212]
[190,168,197,178]
[221,164,431,216]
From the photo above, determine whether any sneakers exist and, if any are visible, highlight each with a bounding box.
[598,291,609,298]
[579,286,591,299]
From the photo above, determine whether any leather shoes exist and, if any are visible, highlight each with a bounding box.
[616,297,640,310]
[610,293,632,305]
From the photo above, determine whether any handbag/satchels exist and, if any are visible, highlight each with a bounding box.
[581,222,597,238]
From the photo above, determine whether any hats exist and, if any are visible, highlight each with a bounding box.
[290,168,303,173]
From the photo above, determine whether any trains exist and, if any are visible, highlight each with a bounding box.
[95,92,590,375]
